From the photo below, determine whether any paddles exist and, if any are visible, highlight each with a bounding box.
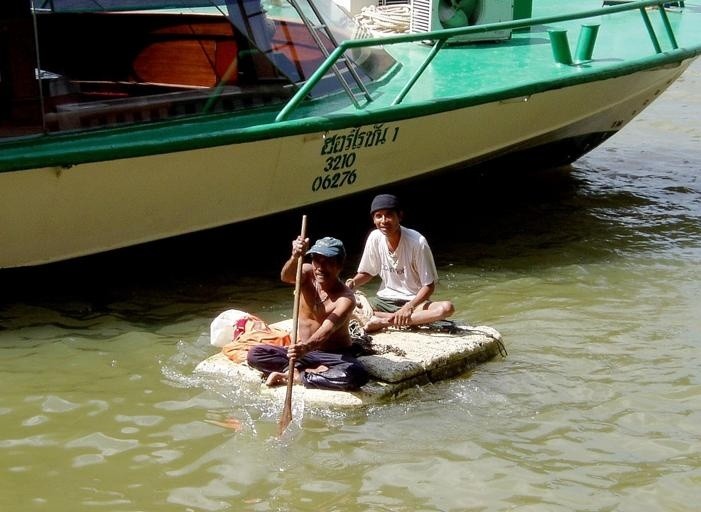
[280,212,306,443]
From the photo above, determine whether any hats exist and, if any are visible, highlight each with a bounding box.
[370,194,397,213]
[305,237,347,260]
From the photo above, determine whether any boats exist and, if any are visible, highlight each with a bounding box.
[0,0,701,297]
[191,313,510,414]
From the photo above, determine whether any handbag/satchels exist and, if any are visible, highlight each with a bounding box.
[223,328,291,363]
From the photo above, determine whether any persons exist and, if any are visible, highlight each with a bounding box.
[246,235,369,390]
[345,193,457,335]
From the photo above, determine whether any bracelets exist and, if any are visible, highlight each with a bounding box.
[346,278,356,288]
[404,302,417,311]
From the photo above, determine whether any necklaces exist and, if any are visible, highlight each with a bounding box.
[313,282,335,306]
